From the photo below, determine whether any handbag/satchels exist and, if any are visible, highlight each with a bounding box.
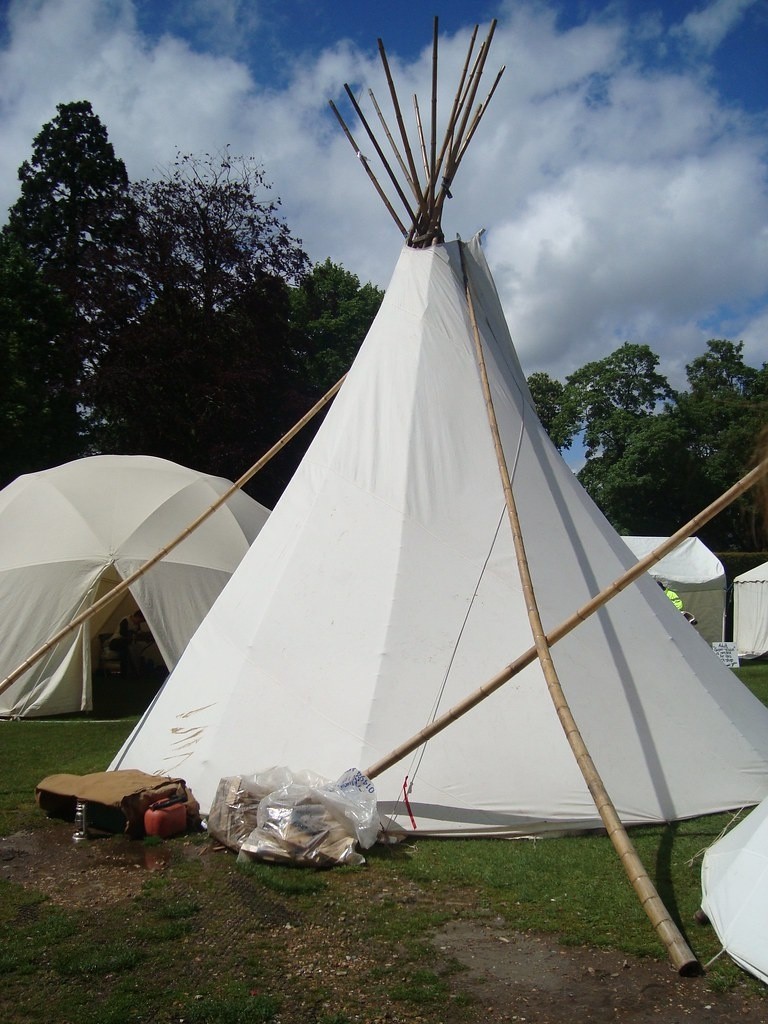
[34,769,202,835]
[208,766,378,867]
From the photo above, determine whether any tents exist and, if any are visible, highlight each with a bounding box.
[0,452,273,719]
[618,536,767,982]
[102,234,767,844]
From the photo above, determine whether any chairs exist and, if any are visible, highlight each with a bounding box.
[98,633,136,679]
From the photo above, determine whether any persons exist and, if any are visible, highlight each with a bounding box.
[107,609,154,681]
